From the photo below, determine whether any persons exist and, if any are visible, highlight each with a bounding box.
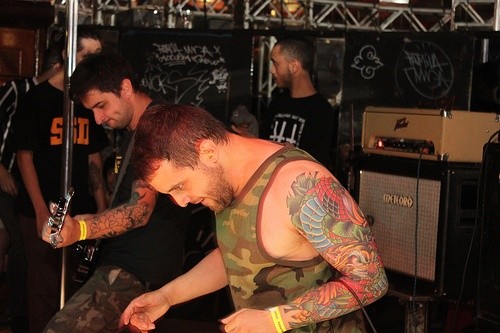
[0,42,67,332]
[6,24,108,333]
[41,44,201,332]
[116,103,389,333]
[231,37,334,161]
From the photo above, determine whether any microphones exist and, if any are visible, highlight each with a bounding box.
[366,215,374,225]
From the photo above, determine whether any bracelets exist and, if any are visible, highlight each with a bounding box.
[270,306,287,332]
[79,220,88,241]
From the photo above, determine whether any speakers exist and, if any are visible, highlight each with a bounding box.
[353,164,481,294]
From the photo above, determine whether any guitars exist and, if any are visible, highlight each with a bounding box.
[48,190,105,282]
